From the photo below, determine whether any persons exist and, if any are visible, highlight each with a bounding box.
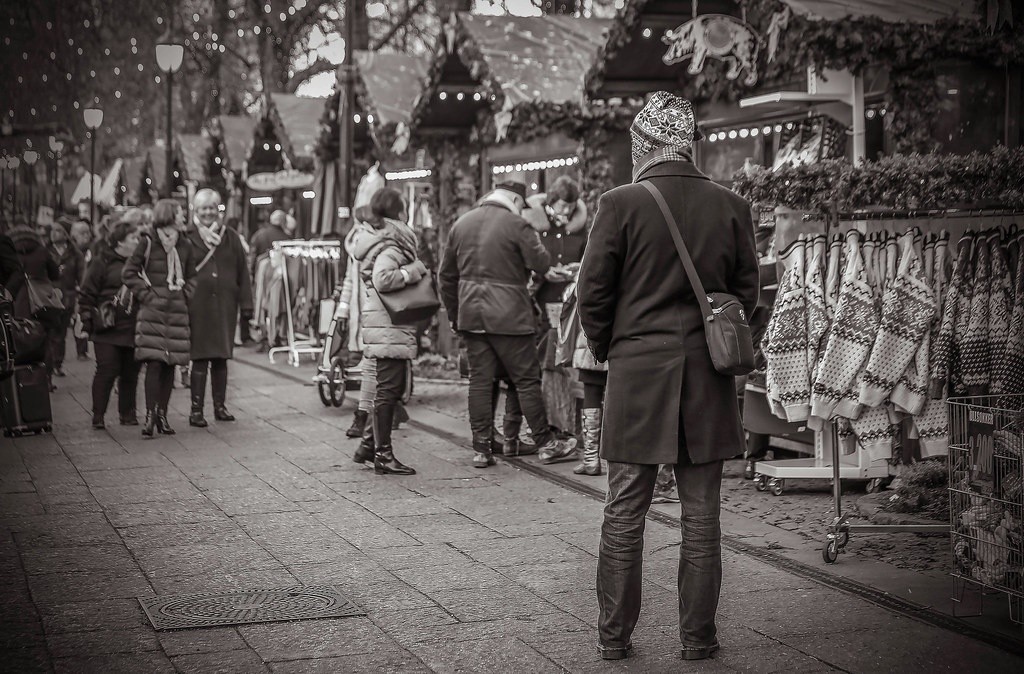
[0,188,298,438]
[578,92,761,659]
[332,174,684,504]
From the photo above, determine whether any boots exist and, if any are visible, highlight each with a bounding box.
[372,403,417,475]
[346,408,369,438]
[141,370,235,436]
[390,399,403,430]
[572,407,608,475]
[353,412,378,464]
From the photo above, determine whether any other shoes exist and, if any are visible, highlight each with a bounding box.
[50,352,91,391]
[92,414,106,429]
[681,636,720,660]
[596,638,633,659]
[119,412,139,425]
[650,463,680,503]
[472,431,578,467]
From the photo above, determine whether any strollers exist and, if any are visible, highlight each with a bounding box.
[313,303,413,406]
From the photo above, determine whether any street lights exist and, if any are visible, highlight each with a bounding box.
[82,94,104,236]
[156,23,184,199]
[1,130,64,224]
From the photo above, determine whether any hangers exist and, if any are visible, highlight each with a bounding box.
[794,206,1024,249]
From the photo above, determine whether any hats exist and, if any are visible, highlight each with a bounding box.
[555,175,578,202]
[54,219,72,241]
[629,90,694,165]
[495,180,532,210]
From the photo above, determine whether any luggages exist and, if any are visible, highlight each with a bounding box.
[0,313,53,438]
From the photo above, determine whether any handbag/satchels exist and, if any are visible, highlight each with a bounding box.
[370,244,441,325]
[91,300,115,331]
[26,277,66,316]
[0,317,46,367]
[119,235,151,313]
[702,292,755,375]
